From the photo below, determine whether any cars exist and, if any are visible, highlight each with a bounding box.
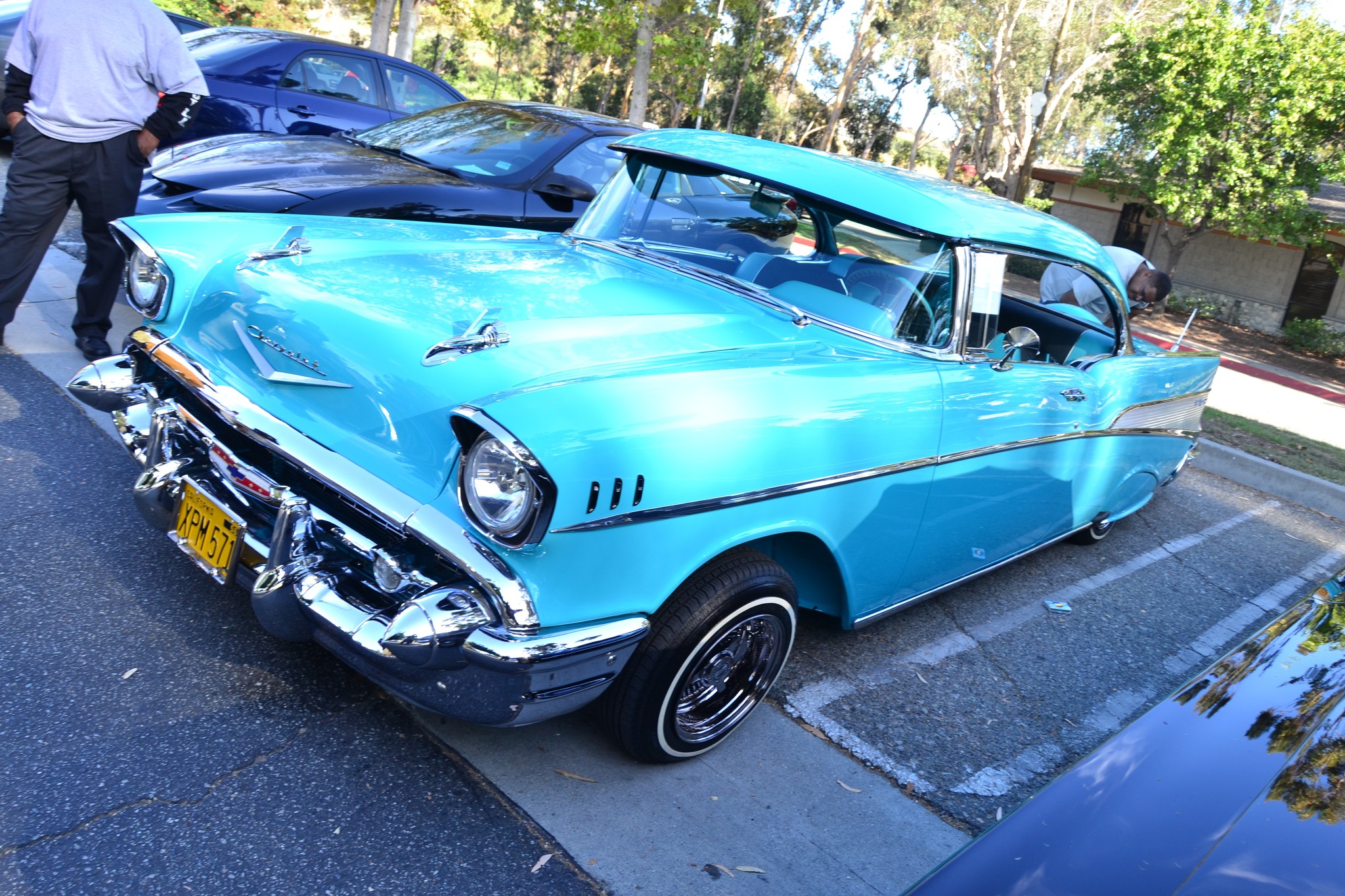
[0,0,517,150]
[134,85,799,255]
[64,127,1220,764]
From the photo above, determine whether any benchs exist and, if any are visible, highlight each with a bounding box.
[829,251,1108,364]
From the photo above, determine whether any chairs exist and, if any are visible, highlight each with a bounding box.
[336,76,362,100]
[734,254,882,308]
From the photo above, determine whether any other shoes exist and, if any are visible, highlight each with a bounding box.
[75,335,112,362]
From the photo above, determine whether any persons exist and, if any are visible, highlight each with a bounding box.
[1039,245,1170,329]
[0,0,210,362]
